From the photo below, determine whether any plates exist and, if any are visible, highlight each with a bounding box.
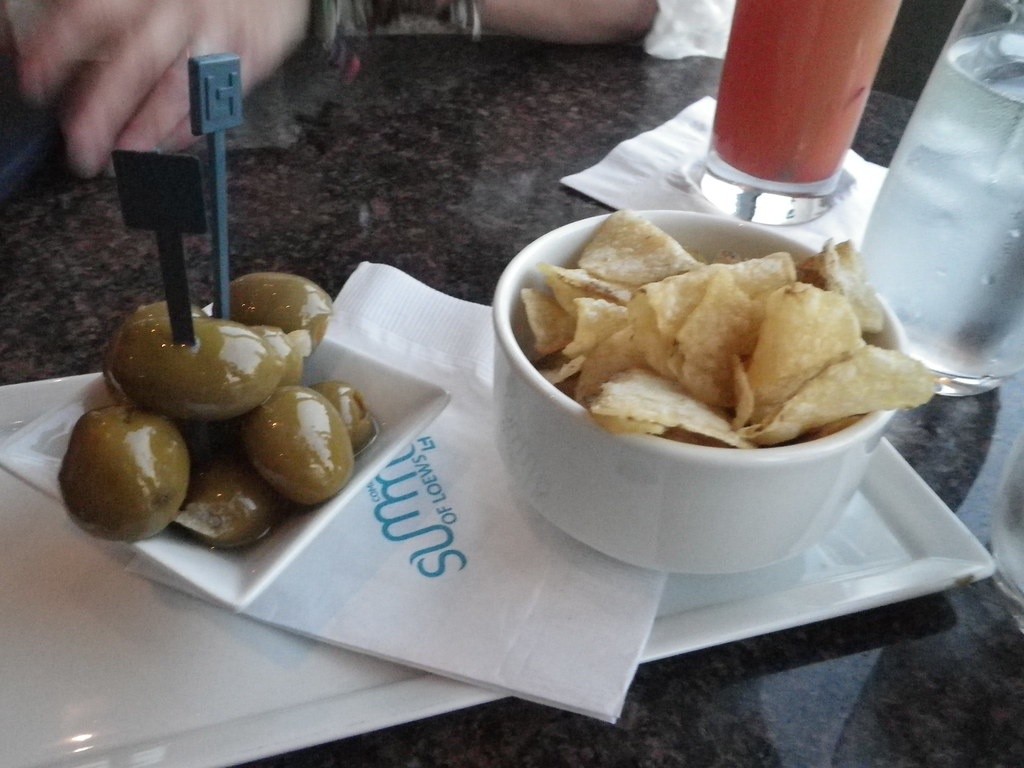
[0,299,452,611]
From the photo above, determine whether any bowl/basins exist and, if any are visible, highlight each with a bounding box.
[492,210,908,577]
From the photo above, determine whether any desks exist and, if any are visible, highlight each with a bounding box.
[0,31,1024,768]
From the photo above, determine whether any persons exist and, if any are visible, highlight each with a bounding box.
[0,0,661,180]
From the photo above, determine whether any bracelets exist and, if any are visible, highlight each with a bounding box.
[314,0,481,43]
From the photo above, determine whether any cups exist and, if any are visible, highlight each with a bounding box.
[700,1,904,225]
[859,1,1024,397]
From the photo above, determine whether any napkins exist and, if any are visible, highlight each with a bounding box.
[560,96,889,254]
[123,260,672,725]
